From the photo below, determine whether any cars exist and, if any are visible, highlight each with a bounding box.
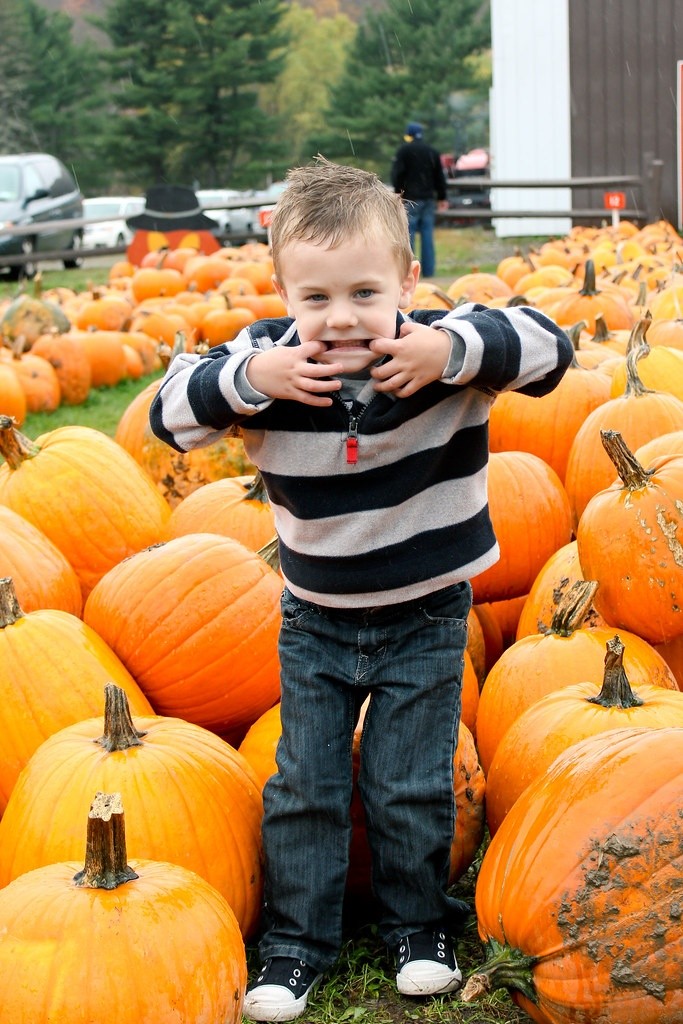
[195,189,247,246]
[0,153,85,280]
[81,196,147,251]
[240,181,285,242]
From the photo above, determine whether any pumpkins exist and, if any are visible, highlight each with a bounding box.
[0,221,683,1024]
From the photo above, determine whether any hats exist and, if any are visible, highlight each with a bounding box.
[409,126,424,140]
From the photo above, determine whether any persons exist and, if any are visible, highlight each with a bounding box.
[148,154,574,1022]
[389,122,450,278]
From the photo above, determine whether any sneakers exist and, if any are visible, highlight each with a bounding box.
[393,927,463,997]
[243,954,324,1023]
